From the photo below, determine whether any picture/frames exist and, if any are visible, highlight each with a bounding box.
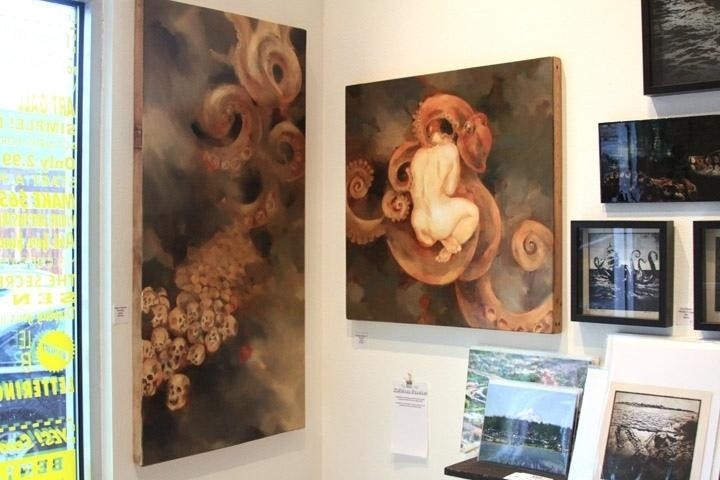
[640,1,719,96]
[594,382,720,480]
[569,220,676,337]
[692,220,720,331]
